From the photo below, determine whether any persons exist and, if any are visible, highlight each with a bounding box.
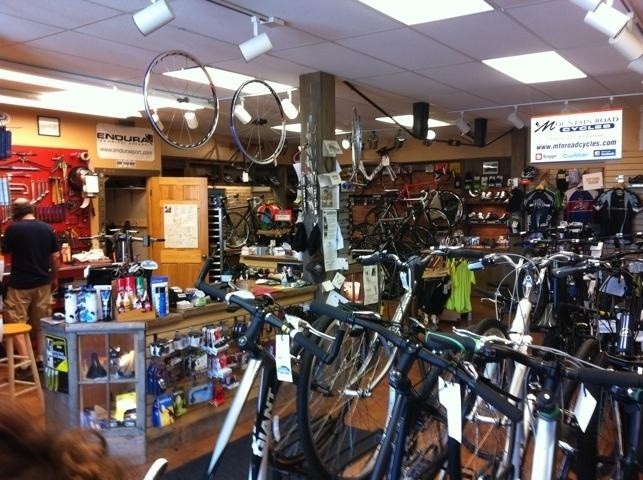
[0,197,61,379]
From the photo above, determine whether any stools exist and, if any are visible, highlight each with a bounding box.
[0,323,44,409]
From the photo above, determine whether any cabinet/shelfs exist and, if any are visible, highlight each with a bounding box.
[41,248,385,469]
[464,191,513,257]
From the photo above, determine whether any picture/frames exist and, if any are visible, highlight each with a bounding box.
[37,116,60,138]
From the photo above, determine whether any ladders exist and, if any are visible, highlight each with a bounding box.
[208,208,223,285]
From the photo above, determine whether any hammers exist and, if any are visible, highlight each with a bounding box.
[48,176,61,204]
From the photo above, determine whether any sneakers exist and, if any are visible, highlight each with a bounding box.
[13,360,45,381]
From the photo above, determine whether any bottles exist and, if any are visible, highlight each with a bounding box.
[64,284,98,324]
[156,287,166,316]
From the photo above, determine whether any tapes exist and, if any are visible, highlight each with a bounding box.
[80,152,89,161]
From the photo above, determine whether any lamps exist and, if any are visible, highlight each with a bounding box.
[131,0,284,64]
[341,102,525,152]
[149,89,296,133]
[568,0,643,76]
